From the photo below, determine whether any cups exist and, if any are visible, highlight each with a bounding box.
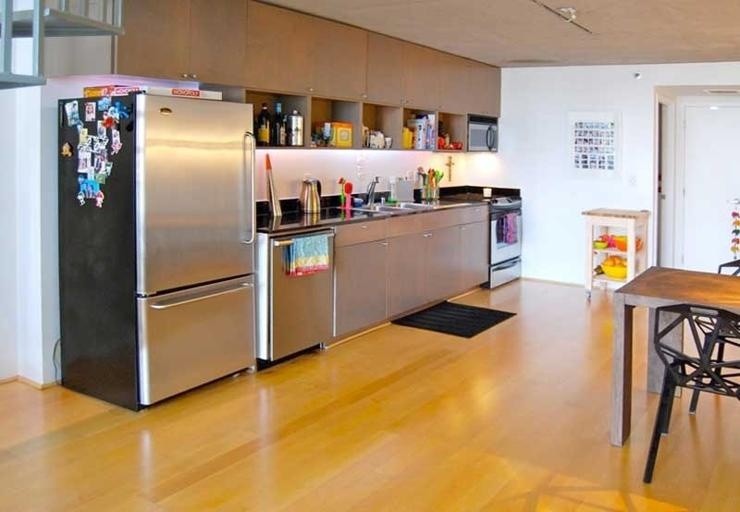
[385,137,392,148]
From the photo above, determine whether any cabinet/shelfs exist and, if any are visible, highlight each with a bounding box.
[581,206,652,304]
[120,1,247,87]
[334,207,490,336]
[248,1,502,153]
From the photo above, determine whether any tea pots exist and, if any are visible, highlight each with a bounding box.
[299,178,322,214]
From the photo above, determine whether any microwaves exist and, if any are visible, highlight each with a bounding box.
[467,121,497,152]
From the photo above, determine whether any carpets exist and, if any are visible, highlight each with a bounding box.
[390,299,517,339]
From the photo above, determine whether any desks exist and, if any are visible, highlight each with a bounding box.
[609,264,740,447]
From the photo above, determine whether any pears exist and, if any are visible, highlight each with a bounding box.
[604,255,627,267]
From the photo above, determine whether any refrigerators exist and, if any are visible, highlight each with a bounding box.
[58,93,256,412]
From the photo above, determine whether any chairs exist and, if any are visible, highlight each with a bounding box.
[642,303,739,483]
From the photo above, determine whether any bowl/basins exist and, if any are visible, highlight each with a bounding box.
[600,264,627,280]
[611,235,627,252]
[593,241,608,249]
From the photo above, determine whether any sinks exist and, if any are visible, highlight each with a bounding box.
[380,201,435,209]
[336,205,410,216]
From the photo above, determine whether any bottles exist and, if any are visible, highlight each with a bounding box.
[257,102,303,147]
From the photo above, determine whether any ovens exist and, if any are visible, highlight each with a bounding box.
[490,208,522,289]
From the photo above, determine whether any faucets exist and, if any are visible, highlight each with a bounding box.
[361,176,383,210]
